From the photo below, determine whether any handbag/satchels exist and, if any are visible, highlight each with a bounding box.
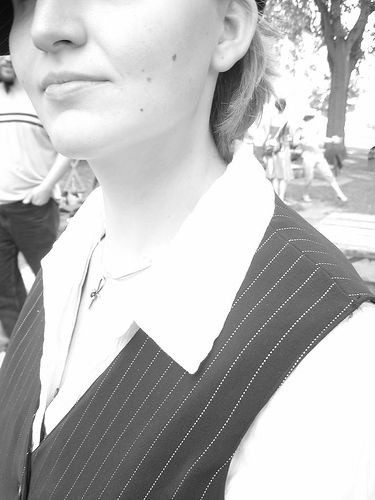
[263,139,282,155]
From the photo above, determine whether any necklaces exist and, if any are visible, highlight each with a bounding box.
[83,259,155,311]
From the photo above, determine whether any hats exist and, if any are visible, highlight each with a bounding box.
[0,0,269,56]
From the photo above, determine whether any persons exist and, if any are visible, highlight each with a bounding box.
[0,0,375,500]
[1,53,72,342]
[263,101,351,206]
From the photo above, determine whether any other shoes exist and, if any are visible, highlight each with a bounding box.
[282,200,295,206]
[337,194,348,203]
[301,195,312,202]
